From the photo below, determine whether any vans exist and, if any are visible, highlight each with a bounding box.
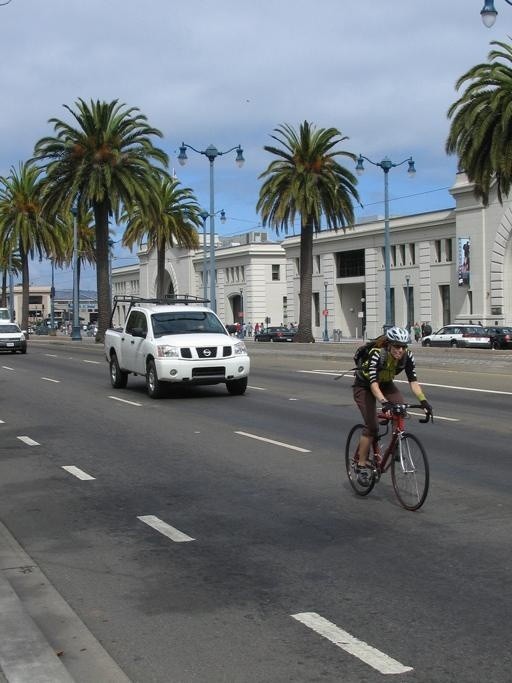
[484,325,512,351]
[421,323,491,348]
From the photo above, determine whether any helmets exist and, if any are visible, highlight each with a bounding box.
[387,326,409,347]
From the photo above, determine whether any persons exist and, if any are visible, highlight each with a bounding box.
[352,327,432,475]
[280,322,297,329]
[233,322,263,341]
[490,329,498,350]
[406,322,432,347]
[462,242,469,270]
[478,321,483,326]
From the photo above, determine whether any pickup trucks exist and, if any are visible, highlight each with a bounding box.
[103,292,251,399]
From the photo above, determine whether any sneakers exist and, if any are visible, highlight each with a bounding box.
[353,462,370,486]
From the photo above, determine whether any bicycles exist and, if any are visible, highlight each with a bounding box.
[343,402,434,512]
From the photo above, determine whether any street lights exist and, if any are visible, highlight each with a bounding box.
[182,204,227,308]
[357,152,417,336]
[177,140,245,314]
[323,281,330,340]
[239,287,244,339]
[404,274,411,339]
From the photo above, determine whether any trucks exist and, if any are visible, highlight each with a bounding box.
[0,307,18,324]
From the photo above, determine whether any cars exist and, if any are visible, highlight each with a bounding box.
[31,316,98,338]
[0,322,28,355]
[255,325,296,342]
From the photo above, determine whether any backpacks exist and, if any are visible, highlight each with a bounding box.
[354,337,407,373]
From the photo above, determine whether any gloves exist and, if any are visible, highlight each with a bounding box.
[382,402,393,414]
[421,400,431,410]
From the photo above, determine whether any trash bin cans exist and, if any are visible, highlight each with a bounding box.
[333,328,340,342]
